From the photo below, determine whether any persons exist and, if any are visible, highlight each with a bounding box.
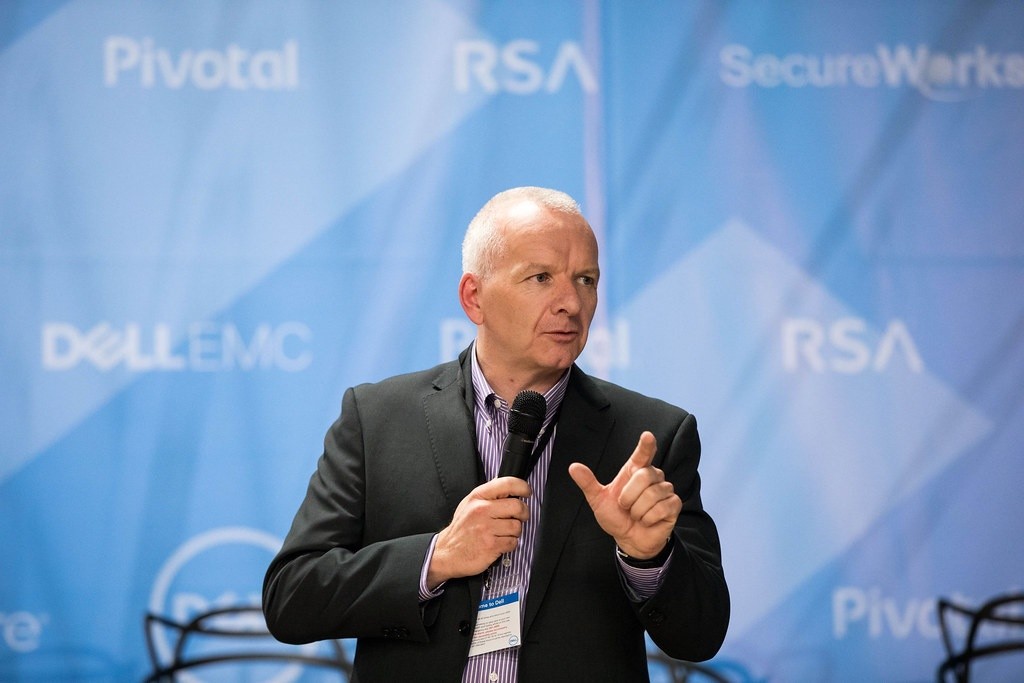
[261,186,731,683]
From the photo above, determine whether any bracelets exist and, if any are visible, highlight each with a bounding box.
[618,537,673,569]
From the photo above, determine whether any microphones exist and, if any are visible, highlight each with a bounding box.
[491,390,547,566]
